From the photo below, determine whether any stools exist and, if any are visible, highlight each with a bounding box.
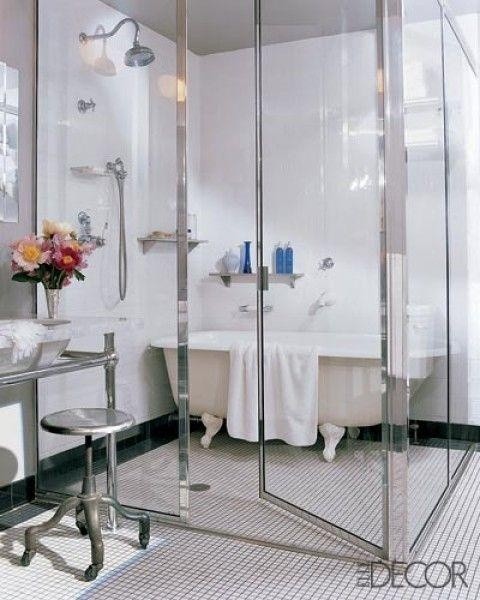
[20,408,152,582]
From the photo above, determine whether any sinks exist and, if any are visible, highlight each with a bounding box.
[0,328,74,377]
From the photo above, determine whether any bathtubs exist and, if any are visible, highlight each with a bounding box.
[151,328,460,430]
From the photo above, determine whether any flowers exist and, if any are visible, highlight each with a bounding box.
[8,215,97,290]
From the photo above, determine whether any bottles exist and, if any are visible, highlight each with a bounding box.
[276,241,294,274]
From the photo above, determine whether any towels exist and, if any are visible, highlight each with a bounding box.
[225,338,318,446]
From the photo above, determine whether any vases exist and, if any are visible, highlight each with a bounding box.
[45,287,60,319]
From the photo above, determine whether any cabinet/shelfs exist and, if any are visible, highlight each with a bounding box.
[0,332,118,530]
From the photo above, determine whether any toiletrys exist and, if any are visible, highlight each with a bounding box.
[285,242,294,274]
[285,241,293,274]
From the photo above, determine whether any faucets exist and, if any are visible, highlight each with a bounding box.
[320,258,335,270]
